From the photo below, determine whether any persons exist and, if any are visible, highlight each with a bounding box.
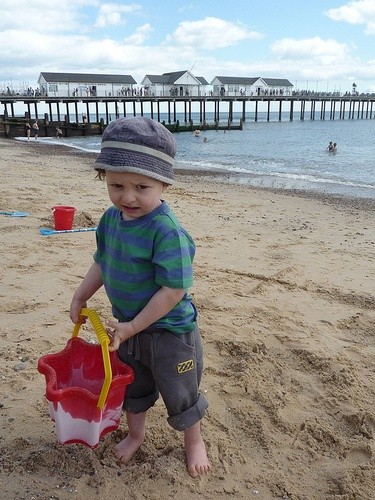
[70,117,211,478]
[0,86,340,158]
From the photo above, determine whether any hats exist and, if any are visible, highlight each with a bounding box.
[93,117,176,186]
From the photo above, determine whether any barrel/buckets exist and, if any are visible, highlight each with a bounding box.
[51,206,76,230]
[38,308,135,449]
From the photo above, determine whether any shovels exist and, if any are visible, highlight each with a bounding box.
[1,212,29,217]
